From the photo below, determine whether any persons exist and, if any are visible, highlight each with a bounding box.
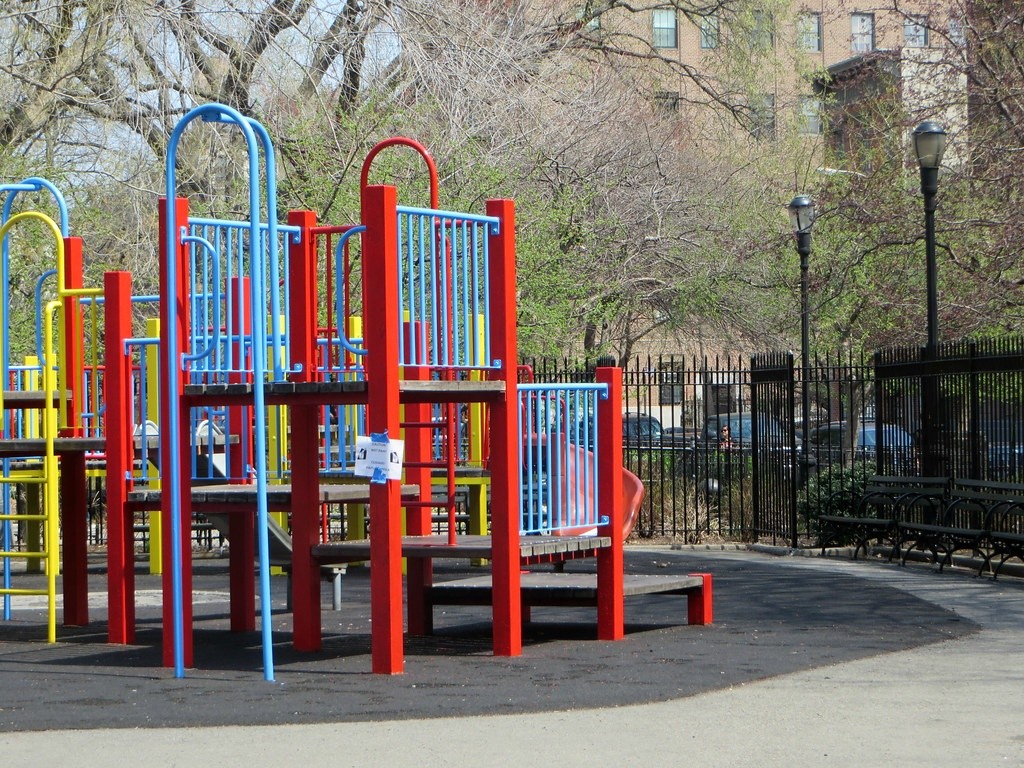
[719,425,740,448]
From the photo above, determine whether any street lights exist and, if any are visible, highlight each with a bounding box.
[913,119,949,345]
[785,194,829,487]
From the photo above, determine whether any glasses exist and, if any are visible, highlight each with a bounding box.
[720,429,729,433]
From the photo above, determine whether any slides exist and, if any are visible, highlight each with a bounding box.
[524,431,645,541]
[136,418,349,574]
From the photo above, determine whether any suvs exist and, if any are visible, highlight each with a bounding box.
[624,411,922,476]
